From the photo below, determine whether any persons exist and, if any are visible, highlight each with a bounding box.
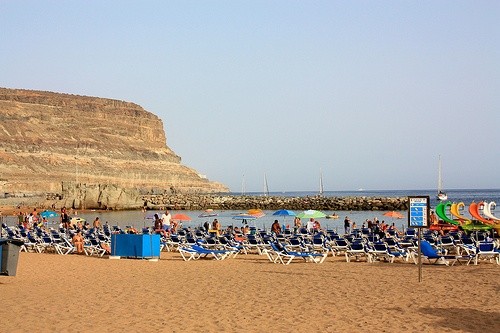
[227,225,251,241]
[154,209,178,237]
[425,226,500,256]
[310,218,321,228]
[333,212,337,215]
[363,217,402,242]
[271,220,281,232]
[430,211,436,223]
[60,206,111,255]
[294,217,302,227]
[18,207,47,230]
[212,219,219,230]
[344,215,357,234]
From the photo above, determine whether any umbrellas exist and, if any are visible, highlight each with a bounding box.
[198,210,219,217]
[143,212,192,220]
[40,210,59,218]
[70,217,86,222]
[272,210,327,219]
[383,210,404,220]
[232,209,264,219]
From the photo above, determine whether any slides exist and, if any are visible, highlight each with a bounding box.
[435,202,456,226]
[449,203,471,225]
[468,202,494,224]
[483,203,500,221]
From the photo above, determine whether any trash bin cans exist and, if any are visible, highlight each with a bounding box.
[0,239,24,275]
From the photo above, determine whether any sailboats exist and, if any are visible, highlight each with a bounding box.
[436,155,448,201]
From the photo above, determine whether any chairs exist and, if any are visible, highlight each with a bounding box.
[0,219,500,268]
